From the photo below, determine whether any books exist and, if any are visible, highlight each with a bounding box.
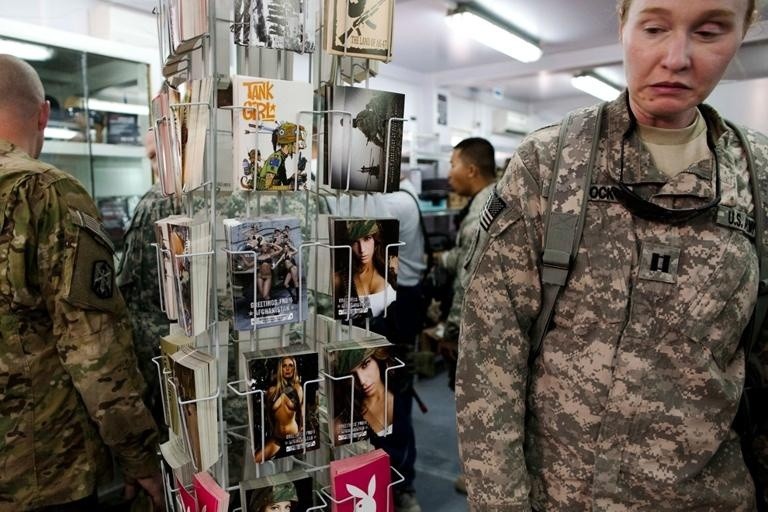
[150,1,410,511]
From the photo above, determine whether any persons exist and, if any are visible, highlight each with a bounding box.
[117,126,187,444]
[0,51,167,512]
[430,136,497,495]
[389,162,429,512]
[457,0,766,512]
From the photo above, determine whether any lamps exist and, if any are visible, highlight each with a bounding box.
[444,7,620,104]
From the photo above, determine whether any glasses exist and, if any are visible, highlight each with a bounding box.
[610,135,721,223]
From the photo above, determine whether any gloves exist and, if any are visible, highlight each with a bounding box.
[297,152,307,185]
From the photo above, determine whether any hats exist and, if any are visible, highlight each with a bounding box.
[251,480,298,507]
[334,348,377,375]
[346,220,378,243]
[276,123,305,144]
